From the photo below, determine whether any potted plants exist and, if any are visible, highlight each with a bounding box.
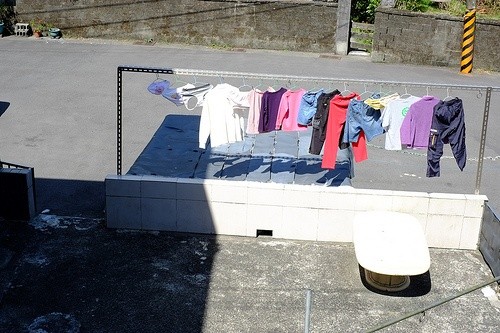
[30,16,61,39]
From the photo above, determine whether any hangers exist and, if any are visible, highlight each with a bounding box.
[271,79,281,88]
[191,75,206,85]
[427,86,431,96]
[400,86,412,97]
[324,81,336,93]
[309,81,321,91]
[355,83,373,99]
[371,84,386,98]
[339,82,352,95]
[255,78,268,88]
[153,73,165,83]
[290,80,300,90]
[168,74,188,87]
[219,76,224,84]
[382,85,396,97]
[239,77,252,89]
[442,87,454,101]
